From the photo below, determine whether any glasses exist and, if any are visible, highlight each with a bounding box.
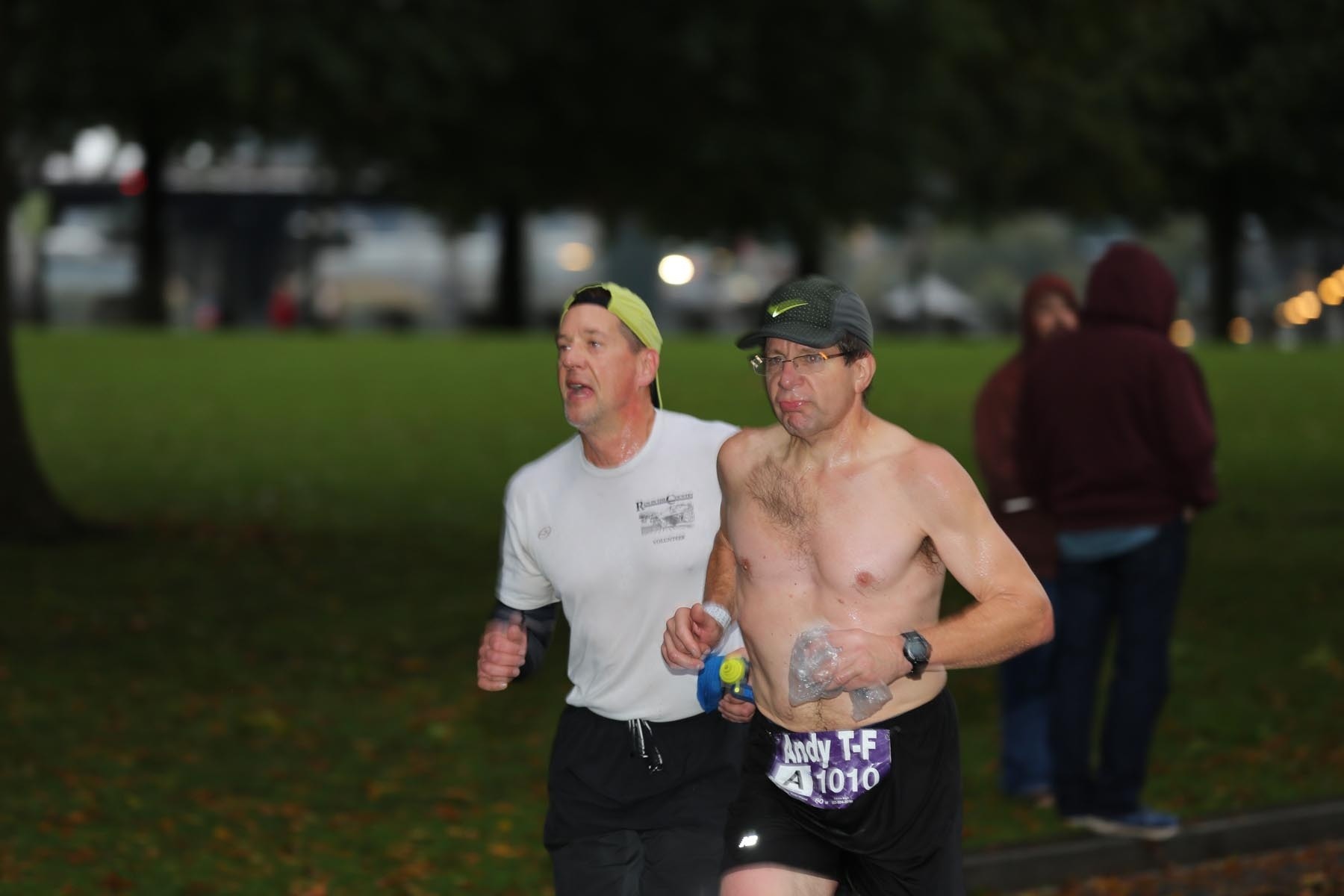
[749,352,845,376]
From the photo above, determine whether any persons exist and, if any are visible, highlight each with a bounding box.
[660,282,1057,896]
[476,284,754,896]
[1022,245,1219,843]
[972,271,1085,811]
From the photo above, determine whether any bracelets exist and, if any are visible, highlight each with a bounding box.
[701,602,736,634]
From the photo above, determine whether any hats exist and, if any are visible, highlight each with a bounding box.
[736,281,874,353]
[559,283,664,410]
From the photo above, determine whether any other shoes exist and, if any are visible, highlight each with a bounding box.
[1065,806,1177,840]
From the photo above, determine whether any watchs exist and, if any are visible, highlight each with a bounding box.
[900,630,931,683]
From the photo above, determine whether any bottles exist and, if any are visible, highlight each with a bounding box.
[719,658,770,727]
